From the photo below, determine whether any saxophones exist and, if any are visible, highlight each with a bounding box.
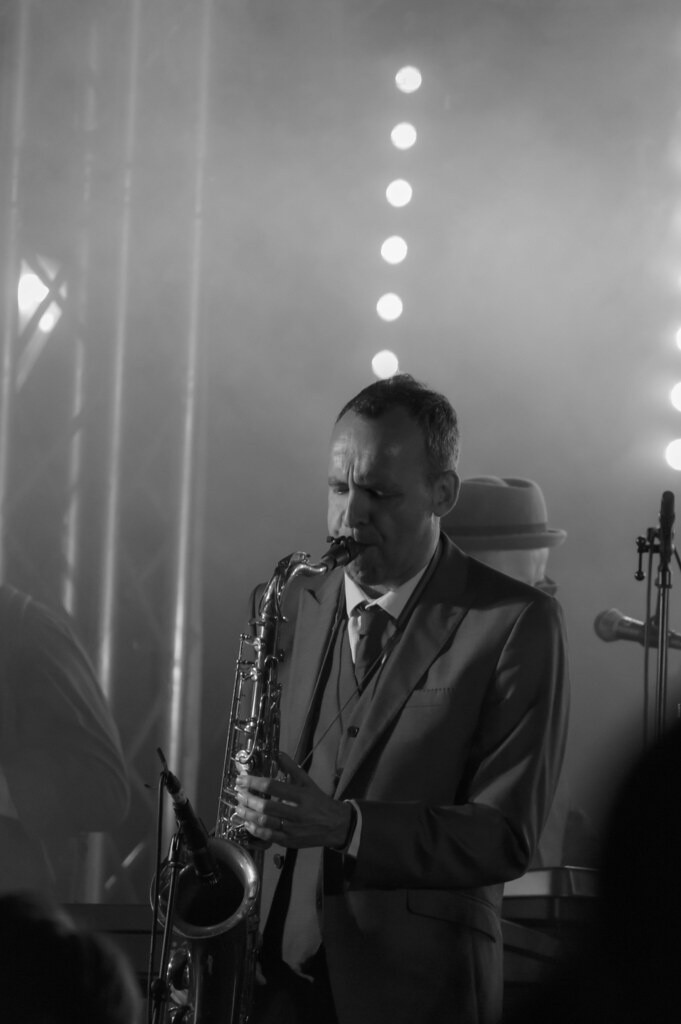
[146,533,368,1024]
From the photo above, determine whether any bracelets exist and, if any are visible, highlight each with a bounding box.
[329,805,357,851]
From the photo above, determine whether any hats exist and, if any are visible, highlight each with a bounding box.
[440,477,568,550]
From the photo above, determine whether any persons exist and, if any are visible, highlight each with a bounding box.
[0,580,131,904]
[235,374,571,1024]
[440,477,567,597]
[0,814,140,1024]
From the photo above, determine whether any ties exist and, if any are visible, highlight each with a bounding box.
[354,604,391,694]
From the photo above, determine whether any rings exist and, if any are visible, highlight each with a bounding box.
[280,818,288,827]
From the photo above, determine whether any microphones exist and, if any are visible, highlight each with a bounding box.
[161,771,222,888]
[593,609,681,652]
